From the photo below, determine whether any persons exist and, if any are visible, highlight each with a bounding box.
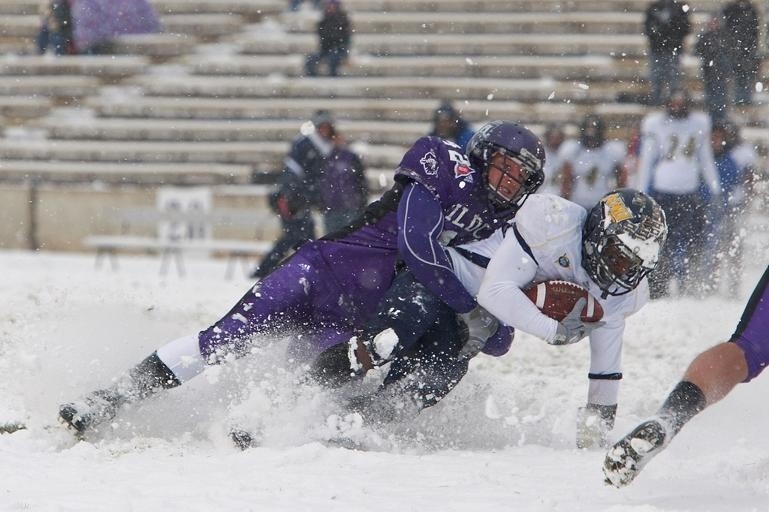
[38,1,769,487]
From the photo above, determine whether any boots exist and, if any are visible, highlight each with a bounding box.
[56,388,122,437]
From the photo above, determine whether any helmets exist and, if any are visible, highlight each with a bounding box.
[581,186,672,288]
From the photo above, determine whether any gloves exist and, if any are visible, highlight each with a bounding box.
[575,398,620,451]
[550,295,608,350]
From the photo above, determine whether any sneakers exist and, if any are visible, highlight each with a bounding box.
[600,416,670,491]
[466,115,552,193]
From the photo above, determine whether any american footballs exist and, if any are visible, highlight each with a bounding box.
[524,282,603,323]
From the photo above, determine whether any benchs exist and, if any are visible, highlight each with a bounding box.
[83,234,279,284]
